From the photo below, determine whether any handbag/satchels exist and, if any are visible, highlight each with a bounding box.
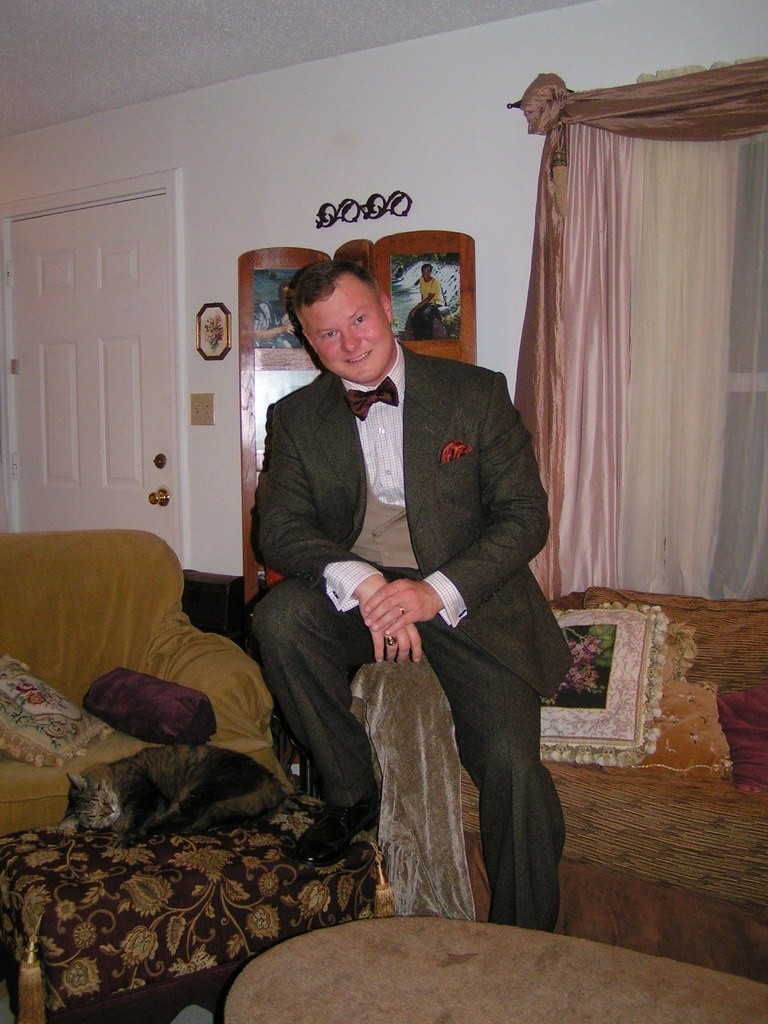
[435,304,449,316]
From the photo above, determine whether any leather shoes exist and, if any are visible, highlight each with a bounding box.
[293,791,381,867]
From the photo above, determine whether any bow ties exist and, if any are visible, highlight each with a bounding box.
[347,376,400,421]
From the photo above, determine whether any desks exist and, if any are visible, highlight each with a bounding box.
[223,916,768,1024]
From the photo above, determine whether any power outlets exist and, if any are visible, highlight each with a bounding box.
[191,392,216,426]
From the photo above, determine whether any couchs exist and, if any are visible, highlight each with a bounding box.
[0,529,296,840]
[341,589,768,983]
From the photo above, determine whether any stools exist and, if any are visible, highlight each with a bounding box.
[0,814,386,1024]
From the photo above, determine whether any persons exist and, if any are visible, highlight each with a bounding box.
[250,261,574,934]
[254,280,304,348]
[405,263,442,341]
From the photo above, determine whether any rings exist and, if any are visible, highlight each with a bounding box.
[399,604,405,615]
[385,636,396,646]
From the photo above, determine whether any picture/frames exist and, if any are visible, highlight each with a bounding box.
[196,302,228,361]
[238,230,477,644]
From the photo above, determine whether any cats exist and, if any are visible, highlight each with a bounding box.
[58,742,286,834]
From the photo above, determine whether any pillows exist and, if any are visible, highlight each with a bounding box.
[539,598,768,796]
[0,651,219,770]
